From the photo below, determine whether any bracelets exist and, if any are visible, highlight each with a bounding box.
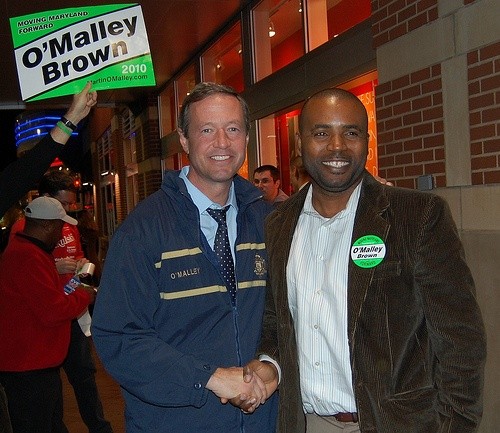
[60,116,78,131]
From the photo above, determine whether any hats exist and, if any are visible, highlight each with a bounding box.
[25,196,78,226]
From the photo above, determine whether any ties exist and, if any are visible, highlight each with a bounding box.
[205,205,236,308]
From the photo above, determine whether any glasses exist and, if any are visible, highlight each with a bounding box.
[253,179,277,185]
[52,199,75,206]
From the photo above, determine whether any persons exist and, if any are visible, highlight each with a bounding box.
[253,165,290,202]
[221,88,488,432]
[11,171,113,432]
[0,194,93,432]
[92,82,280,433]
[1,82,98,207]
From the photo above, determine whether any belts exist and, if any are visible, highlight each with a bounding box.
[333,411,359,423]
